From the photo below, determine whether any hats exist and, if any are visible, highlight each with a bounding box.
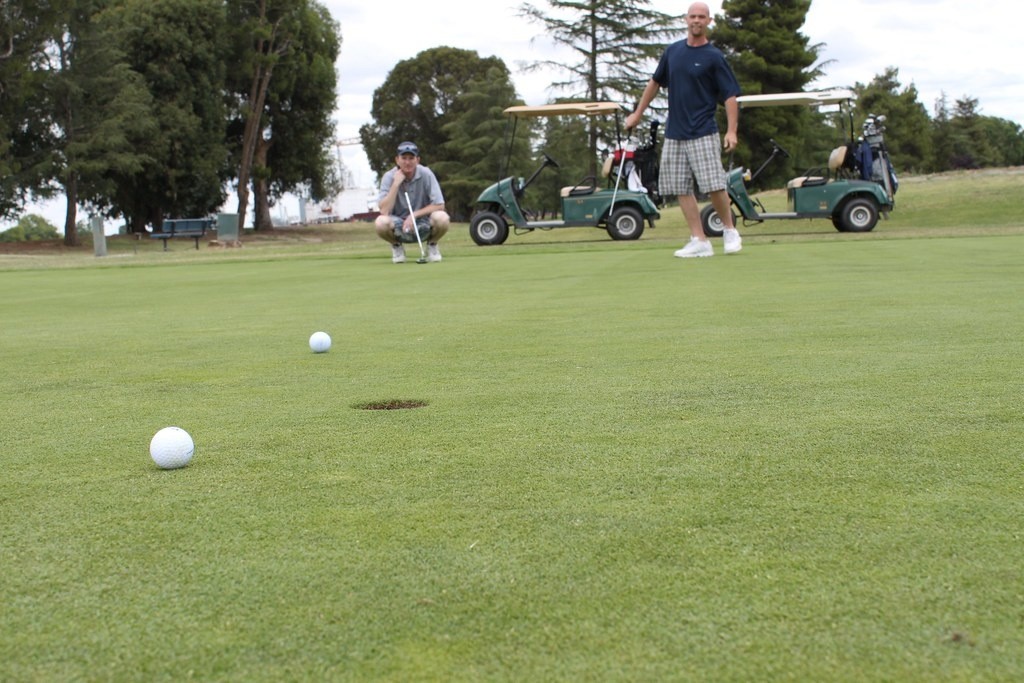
[398,141,419,156]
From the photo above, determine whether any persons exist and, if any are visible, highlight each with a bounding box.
[374,141,450,263]
[623,1,742,259]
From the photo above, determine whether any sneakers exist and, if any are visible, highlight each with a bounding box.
[426,243,442,261]
[392,245,406,263]
[723,229,741,253]
[674,235,714,257]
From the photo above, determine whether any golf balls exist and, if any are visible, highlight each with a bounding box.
[307,331,333,354]
[149,425,195,470]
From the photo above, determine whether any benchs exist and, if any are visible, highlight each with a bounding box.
[149,218,213,252]
[786,146,846,189]
[560,157,614,197]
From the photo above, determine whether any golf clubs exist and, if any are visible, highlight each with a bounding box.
[856,113,888,142]
[603,127,634,227]
[637,136,661,149]
[397,165,431,264]
